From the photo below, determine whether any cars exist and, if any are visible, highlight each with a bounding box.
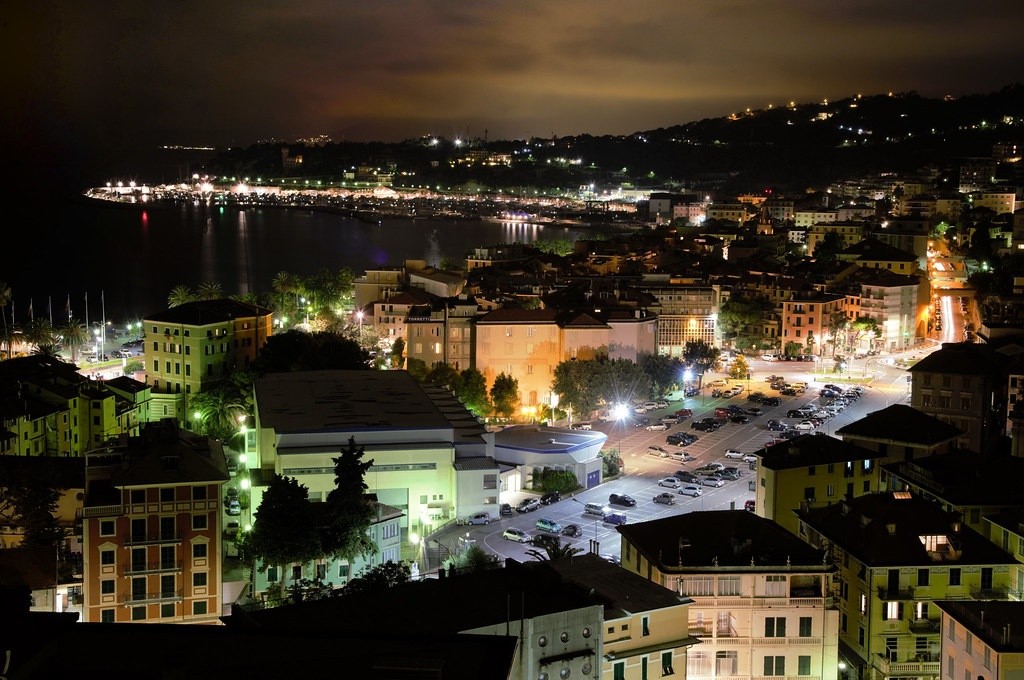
[462,351,863,564]
[219,454,250,562]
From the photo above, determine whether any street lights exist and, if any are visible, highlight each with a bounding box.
[571,498,598,543]
[615,405,626,454]
[680,463,704,511]
[868,385,890,406]
[432,539,456,565]
